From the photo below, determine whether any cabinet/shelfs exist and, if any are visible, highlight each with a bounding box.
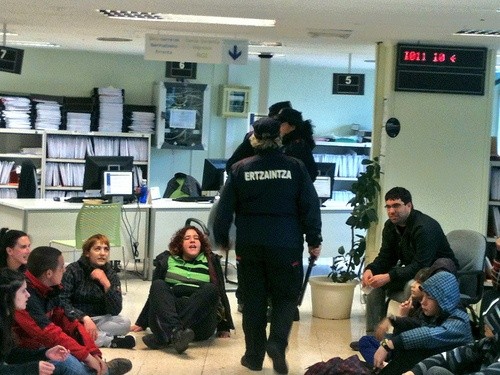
[0,128,45,198]
[45,131,151,198]
[310,143,371,199]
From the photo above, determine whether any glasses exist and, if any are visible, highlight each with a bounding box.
[385,204,405,209]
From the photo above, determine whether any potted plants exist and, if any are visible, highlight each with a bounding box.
[307,154,384,319]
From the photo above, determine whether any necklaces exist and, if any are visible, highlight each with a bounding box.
[186,261,196,279]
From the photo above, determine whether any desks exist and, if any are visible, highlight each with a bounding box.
[148,199,370,281]
[0,198,152,268]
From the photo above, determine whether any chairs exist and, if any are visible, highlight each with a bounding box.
[392,229,486,321]
[48,203,128,295]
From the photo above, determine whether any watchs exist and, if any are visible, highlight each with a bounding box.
[380,339,391,352]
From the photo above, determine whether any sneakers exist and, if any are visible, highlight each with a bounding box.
[104,358,132,375]
[110,335,136,348]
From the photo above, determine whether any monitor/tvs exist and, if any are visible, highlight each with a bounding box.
[201,159,229,191]
[83,156,134,197]
[314,162,335,192]
[312,176,331,208]
[102,171,134,204]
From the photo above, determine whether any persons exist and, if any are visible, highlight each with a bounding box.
[0,101,322,375]
[350,187,500,375]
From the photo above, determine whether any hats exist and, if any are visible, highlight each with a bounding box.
[275,107,302,125]
[253,118,281,139]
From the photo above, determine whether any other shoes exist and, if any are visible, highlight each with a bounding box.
[266,339,288,375]
[142,333,173,349]
[171,328,194,354]
[241,355,263,371]
[350,341,360,351]
